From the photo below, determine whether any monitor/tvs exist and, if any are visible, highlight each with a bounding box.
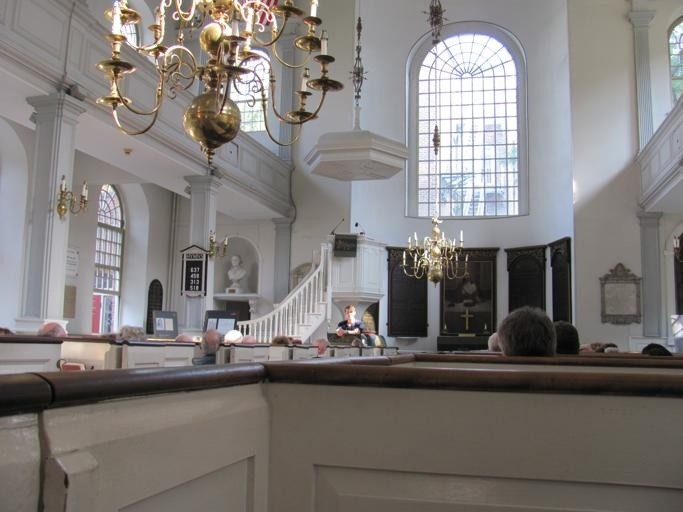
[333,234,358,258]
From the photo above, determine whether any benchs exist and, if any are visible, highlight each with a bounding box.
[0,343,683,512]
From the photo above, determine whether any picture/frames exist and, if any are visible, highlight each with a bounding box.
[598,262,643,325]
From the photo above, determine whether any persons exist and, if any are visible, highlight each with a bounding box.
[583,341,618,355]
[641,343,672,356]
[117,326,146,342]
[176,305,367,365]
[499,306,557,357]
[37,322,68,337]
[489,332,502,352]
[554,321,580,354]
[227,255,247,281]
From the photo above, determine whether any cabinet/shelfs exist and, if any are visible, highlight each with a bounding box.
[331,244,384,295]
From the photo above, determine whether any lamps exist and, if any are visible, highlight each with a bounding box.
[57,174,88,220]
[95,0,344,165]
[400,0,469,287]
[303,17,410,181]
[209,230,229,258]
